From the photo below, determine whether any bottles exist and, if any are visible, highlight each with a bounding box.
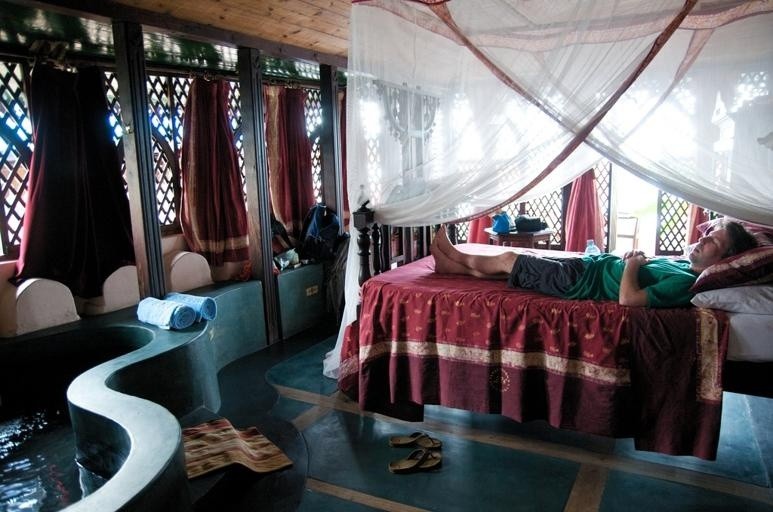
[585,239,601,256]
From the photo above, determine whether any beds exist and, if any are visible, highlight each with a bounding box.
[362,242,773,399]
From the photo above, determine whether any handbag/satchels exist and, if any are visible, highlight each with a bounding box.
[493,211,548,233]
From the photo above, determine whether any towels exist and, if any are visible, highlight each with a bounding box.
[137,292,218,330]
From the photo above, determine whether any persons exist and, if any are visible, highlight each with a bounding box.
[429,221,758,308]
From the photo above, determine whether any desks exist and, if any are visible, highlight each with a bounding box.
[484,226,555,250]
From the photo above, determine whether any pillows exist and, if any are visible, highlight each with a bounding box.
[688,232,772,316]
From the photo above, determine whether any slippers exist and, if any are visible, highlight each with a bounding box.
[388,431,443,473]
